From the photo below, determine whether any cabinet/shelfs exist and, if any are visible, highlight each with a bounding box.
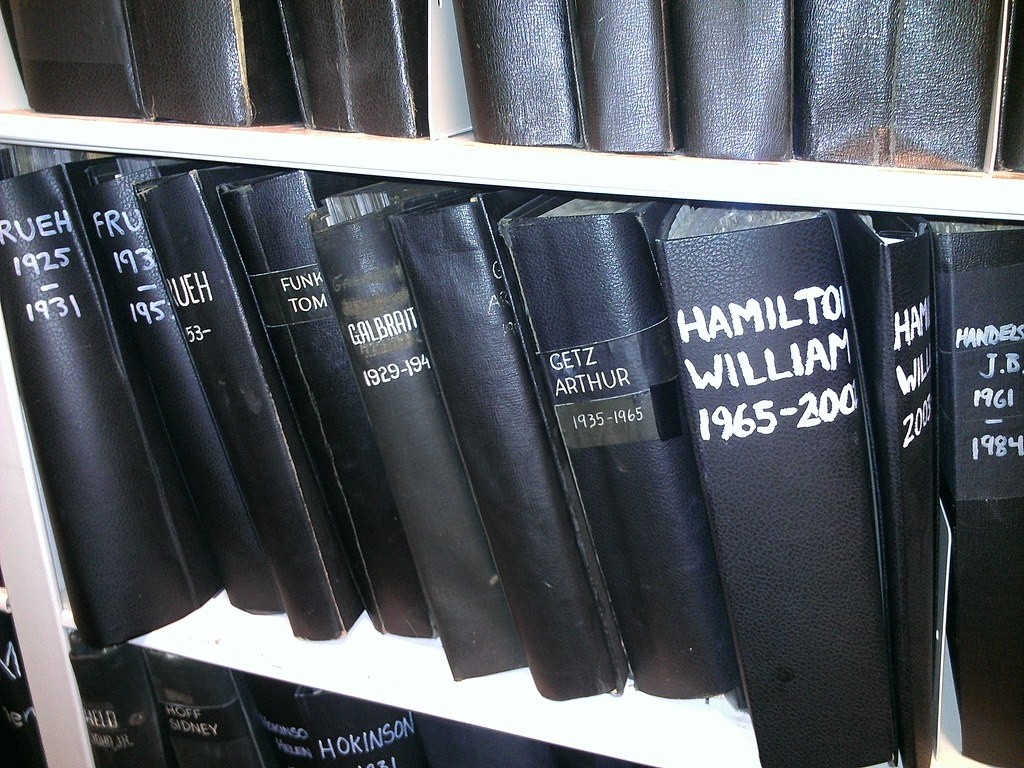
[1,11,1024,768]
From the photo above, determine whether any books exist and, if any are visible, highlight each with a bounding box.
[0,145,1024,768]
[0,0,1024,179]
